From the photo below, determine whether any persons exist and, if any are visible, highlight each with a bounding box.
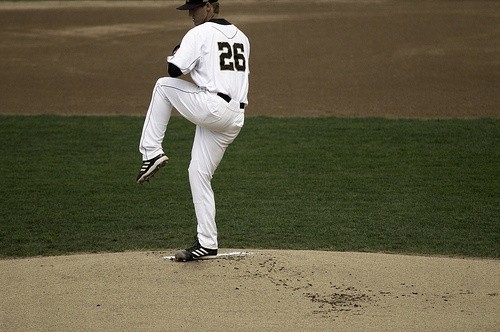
[135,0,252,263]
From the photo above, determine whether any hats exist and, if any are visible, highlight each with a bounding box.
[176,0,218,11]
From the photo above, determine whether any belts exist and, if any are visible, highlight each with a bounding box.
[217,92,245,109]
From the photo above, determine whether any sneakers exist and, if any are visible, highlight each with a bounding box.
[176,241,217,260]
[136,154,168,184]
